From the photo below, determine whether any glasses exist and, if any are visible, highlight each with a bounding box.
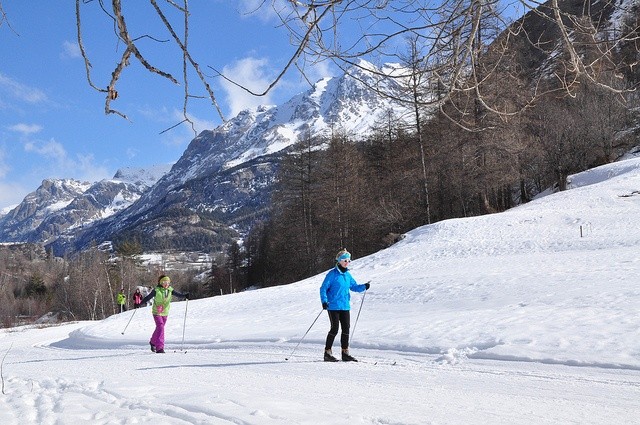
[341,259,351,263]
[162,282,170,284]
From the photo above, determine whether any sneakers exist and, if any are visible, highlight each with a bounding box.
[156,349,165,353]
[150,341,156,352]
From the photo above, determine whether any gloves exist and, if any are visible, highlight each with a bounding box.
[136,301,144,308]
[365,283,370,290]
[322,302,329,310]
[182,292,190,299]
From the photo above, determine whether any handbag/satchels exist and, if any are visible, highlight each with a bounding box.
[154,299,164,313]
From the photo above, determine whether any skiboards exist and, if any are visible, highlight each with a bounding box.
[140,351,188,353]
[308,361,397,365]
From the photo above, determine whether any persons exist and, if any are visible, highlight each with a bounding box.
[137,273,189,355]
[318,248,371,364]
[133,288,143,310]
[116,288,127,313]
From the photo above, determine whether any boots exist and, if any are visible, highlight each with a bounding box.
[342,347,358,361]
[324,347,339,362]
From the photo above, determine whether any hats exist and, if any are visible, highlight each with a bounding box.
[336,247,351,262]
[158,275,171,285]
